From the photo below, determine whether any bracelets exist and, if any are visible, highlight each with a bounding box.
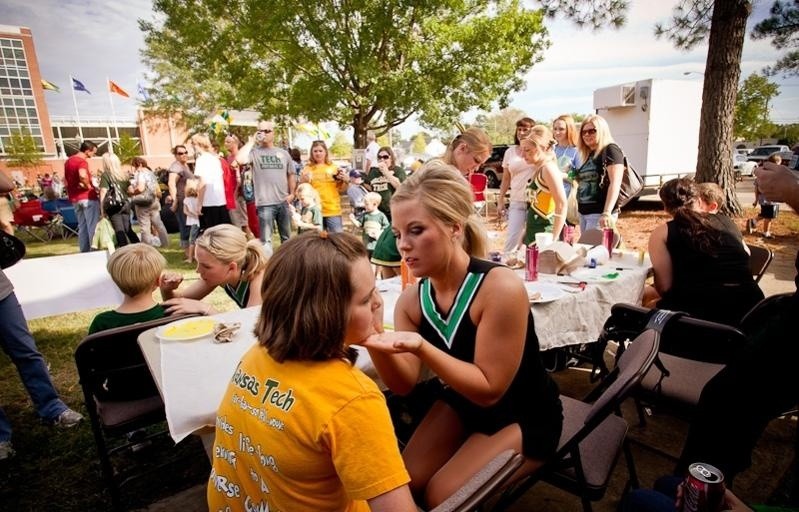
[202,304,213,316]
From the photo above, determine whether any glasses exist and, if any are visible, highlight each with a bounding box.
[581,129,597,135]
[176,151,188,156]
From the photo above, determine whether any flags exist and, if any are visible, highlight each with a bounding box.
[71,77,92,95]
[39,79,64,95]
[136,83,148,103]
[108,80,130,99]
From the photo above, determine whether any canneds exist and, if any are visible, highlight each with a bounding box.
[525,245,540,281]
[681,463,725,512]
[603,227,614,258]
[256,134,265,141]
[564,226,574,246]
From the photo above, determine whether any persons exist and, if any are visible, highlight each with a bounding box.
[750,161,799,412]
[641,180,728,311]
[645,174,766,331]
[237,121,296,258]
[745,154,783,240]
[205,229,421,511]
[610,472,799,512]
[10,173,64,213]
[287,142,407,257]
[167,135,261,262]
[0,167,88,463]
[158,224,279,317]
[370,129,492,281]
[88,242,176,455]
[497,116,624,252]
[348,159,564,511]
[64,140,169,254]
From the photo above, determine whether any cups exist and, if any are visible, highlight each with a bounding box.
[534,232,553,248]
[585,245,609,265]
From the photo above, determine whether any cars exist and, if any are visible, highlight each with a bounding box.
[733,154,758,177]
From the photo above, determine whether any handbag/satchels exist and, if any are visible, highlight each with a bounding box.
[600,163,646,209]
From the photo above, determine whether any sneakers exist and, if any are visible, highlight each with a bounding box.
[747,219,775,239]
[0,440,16,462]
[51,408,86,430]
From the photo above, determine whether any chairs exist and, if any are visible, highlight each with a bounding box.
[58,207,81,242]
[7,217,58,244]
[425,448,524,512]
[513,326,664,504]
[74,312,220,504]
[465,173,490,223]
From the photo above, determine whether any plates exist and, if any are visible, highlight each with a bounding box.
[153,315,215,341]
[509,258,620,302]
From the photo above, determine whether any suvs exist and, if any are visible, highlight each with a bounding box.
[747,145,790,165]
[473,144,515,188]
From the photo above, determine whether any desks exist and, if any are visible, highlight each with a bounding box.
[2,245,124,322]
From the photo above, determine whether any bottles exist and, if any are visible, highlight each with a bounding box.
[257,131,267,141]
